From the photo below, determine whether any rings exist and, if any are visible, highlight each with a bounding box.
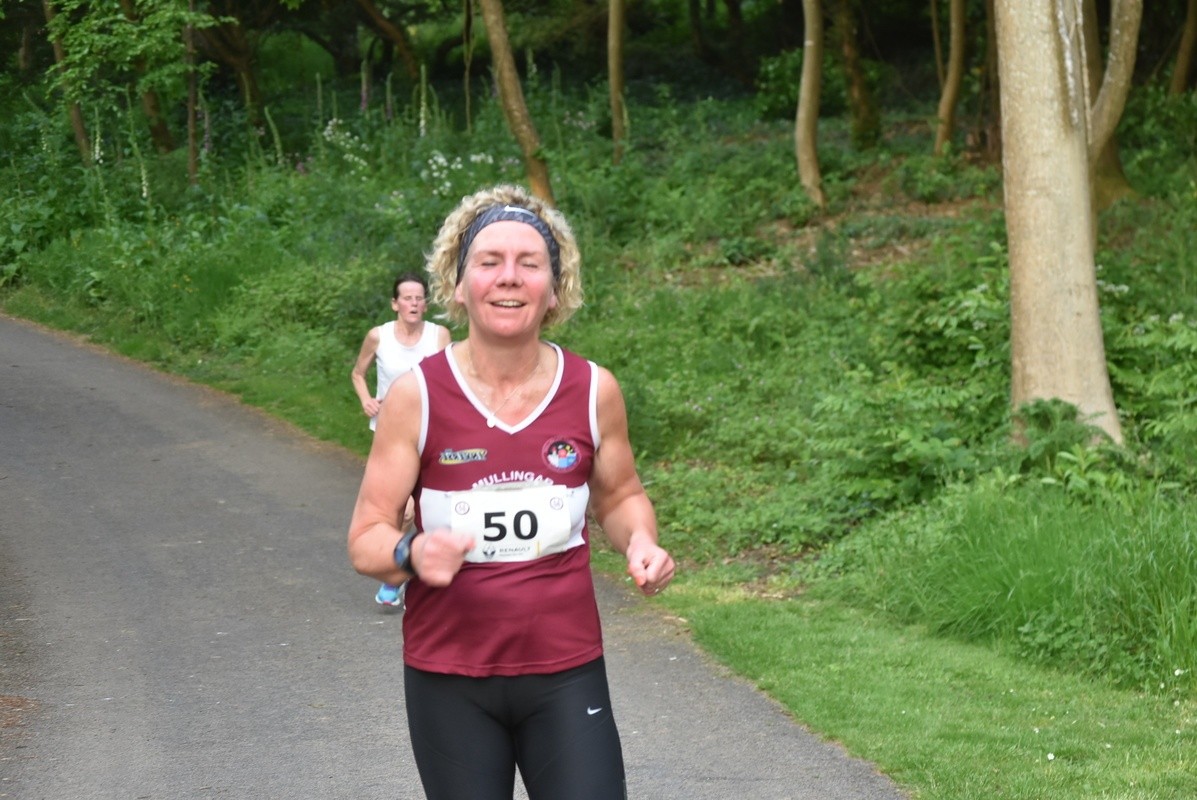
[655,588,659,593]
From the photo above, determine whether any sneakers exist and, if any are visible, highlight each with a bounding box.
[375,580,400,607]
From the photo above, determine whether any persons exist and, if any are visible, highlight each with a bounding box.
[345,183,675,800]
[351,275,452,610]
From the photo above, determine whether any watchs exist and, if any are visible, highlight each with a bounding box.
[393,532,418,574]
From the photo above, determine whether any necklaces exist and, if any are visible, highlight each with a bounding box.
[471,365,535,427]
